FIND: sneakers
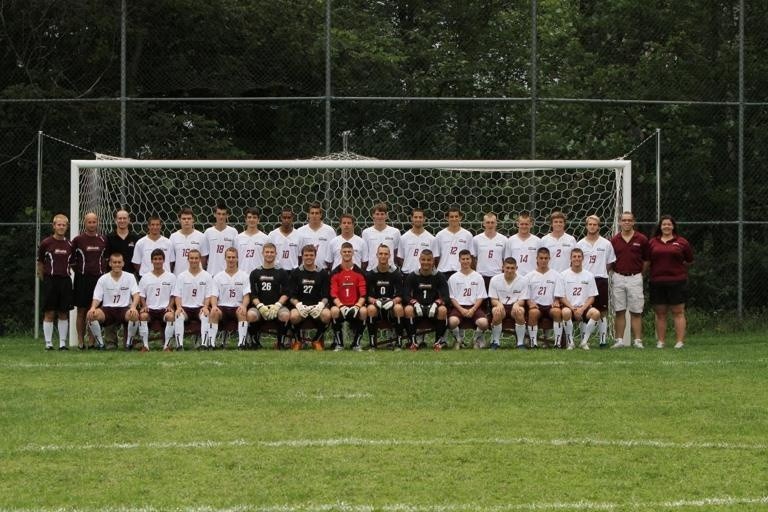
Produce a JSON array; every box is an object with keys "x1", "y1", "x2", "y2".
[
  {"x1": 237, "y1": 343, "x2": 261, "y2": 351},
  {"x1": 196, "y1": 344, "x2": 216, "y2": 352},
  {"x1": 674, "y1": 342, "x2": 685, "y2": 350},
  {"x1": 486, "y1": 343, "x2": 589, "y2": 351},
  {"x1": 633, "y1": 340, "x2": 644, "y2": 350},
  {"x1": 610, "y1": 337, "x2": 624, "y2": 350},
  {"x1": 452, "y1": 340, "x2": 482, "y2": 351},
  {"x1": 58, "y1": 346, "x2": 67, "y2": 352},
  {"x1": 75, "y1": 343, "x2": 119, "y2": 353},
  {"x1": 273, "y1": 337, "x2": 309, "y2": 352},
  {"x1": 312, "y1": 340, "x2": 447, "y2": 354},
  {"x1": 656, "y1": 340, "x2": 664, "y2": 349},
  {"x1": 43, "y1": 345, "x2": 53, "y2": 351},
  {"x1": 123, "y1": 344, "x2": 185, "y2": 354}
]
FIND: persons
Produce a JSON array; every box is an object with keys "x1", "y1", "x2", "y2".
[
  {"x1": 609, "y1": 211, "x2": 651, "y2": 352},
  {"x1": 649, "y1": 215, "x2": 695, "y2": 350},
  {"x1": 70, "y1": 213, "x2": 105, "y2": 351},
  {"x1": 37, "y1": 212, "x2": 74, "y2": 352},
  {"x1": 88, "y1": 202, "x2": 617, "y2": 351}
]
